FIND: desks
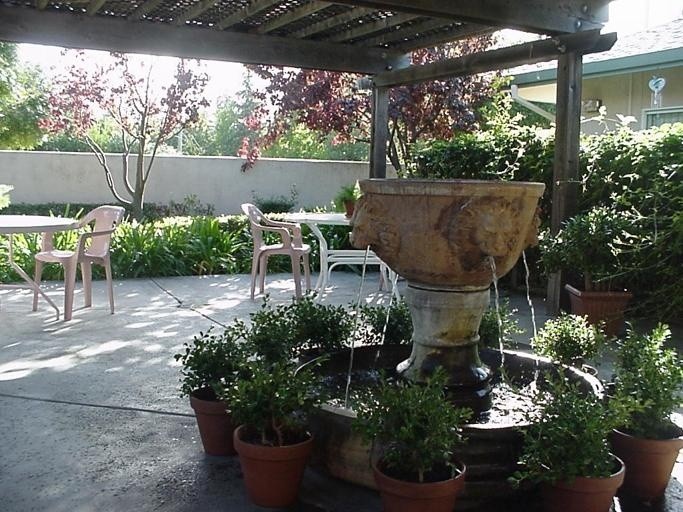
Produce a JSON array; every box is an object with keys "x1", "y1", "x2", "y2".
[
  {"x1": 282, "y1": 210, "x2": 403, "y2": 309},
  {"x1": 0, "y1": 213, "x2": 80, "y2": 323}
]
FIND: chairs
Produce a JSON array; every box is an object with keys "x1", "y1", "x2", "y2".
[
  {"x1": 240, "y1": 201, "x2": 313, "y2": 306},
  {"x1": 30, "y1": 204, "x2": 126, "y2": 321}
]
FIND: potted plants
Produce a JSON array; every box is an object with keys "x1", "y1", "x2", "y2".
[
  {"x1": 341, "y1": 360, "x2": 477, "y2": 512},
  {"x1": 531, "y1": 311, "x2": 602, "y2": 382},
  {"x1": 606, "y1": 321, "x2": 683, "y2": 500},
  {"x1": 211, "y1": 354, "x2": 334, "y2": 508},
  {"x1": 533, "y1": 190, "x2": 648, "y2": 342},
  {"x1": 172, "y1": 323, "x2": 261, "y2": 457},
  {"x1": 497, "y1": 374, "x2": 627, "y2": 512}
]
